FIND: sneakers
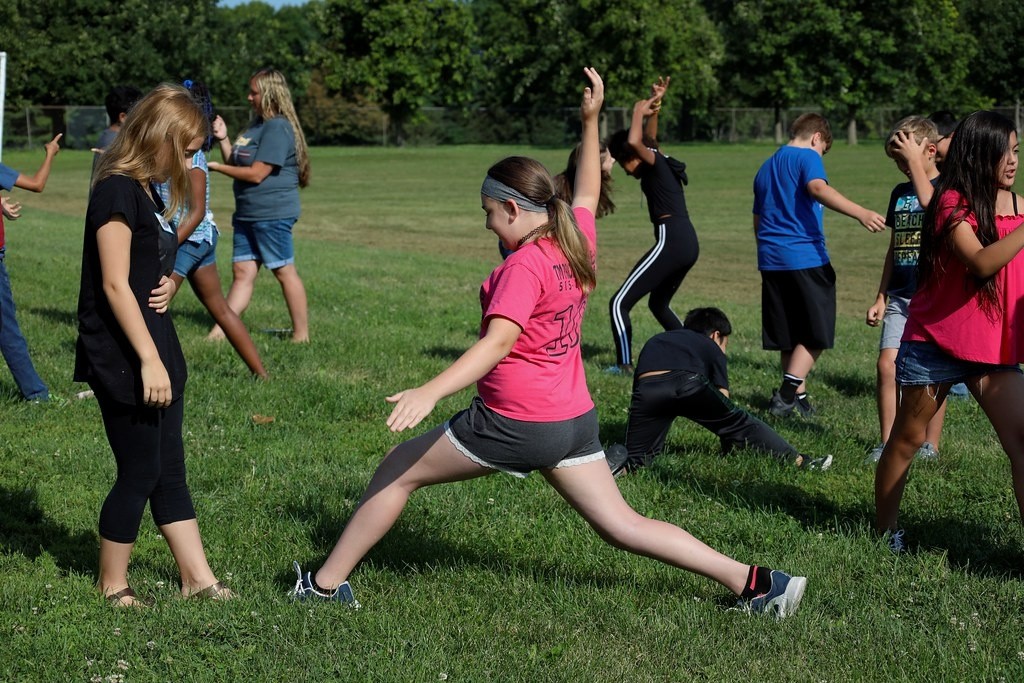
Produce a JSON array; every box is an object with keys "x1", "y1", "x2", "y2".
[
  {"x1": 286, "y1": 560, "x2": 362, "y2": 611},
  {"x1": 604, "y1": 444, "x2": 629, "y2": 480},
  {"x1": 799, "y1": 454, "x2": 833, "y2": 471},
  {"x1": 725, "y1": 570, "x2": 807, "y2": 619},
  {"x1": 768, "y1": 387, "x2": 817, "y2": 421}
]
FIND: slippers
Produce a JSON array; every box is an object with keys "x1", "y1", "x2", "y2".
[
  {"x1": 106, "y1": 587, "x2": 147, "y2": 607},
  {"x1": 188, "y1": 581, "x2": 246, "y2": 601}
]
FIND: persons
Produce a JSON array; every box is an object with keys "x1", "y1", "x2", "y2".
[
  {"x1": 865, "y1": 109, "x2": 1024, "y2": 556},
  {"x1": 603, "y1": 73, "x2": 701, "y2": 373},
  {"x1": 267, "y1": 65, "x2": 808, "y2": 624},
  {"x1": 602, "y1": 306, "x2": 834, "y2": 482},
  {"x1": 752, "y1": 112, "x2": 888, "y2": 420},
  {"x1": 497, "y1": 137, "x2": 615, "y2": 262},
  {"x1": 0, "y1": 132, "x2": 65, "y2": 408},
  {"x1": 69, "y1": 77, "x2": 250, "y2": 620},
  {"x1": 91, "y1": 65, "x2": 309, "y2": 380}
]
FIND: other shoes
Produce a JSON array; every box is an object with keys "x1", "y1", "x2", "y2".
[
  {"x1": 881, "y1": 529, "x2": 906, "y2": 556},
  {"x1": 914, "y1": 442, "x2": 940, "y2": 459},
  {"x1": 604, "y1": 365, "x2": 636, "y2": 373},
  {"x1": 30, "y1": 393, "x2": 67, "y2": 408},
  {"x1": 865, "y1": 443, "x2": 885, "y2": 465}
]
